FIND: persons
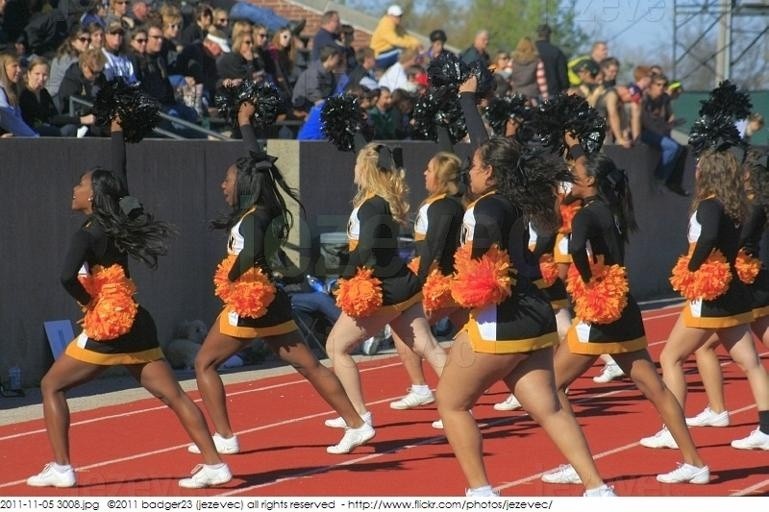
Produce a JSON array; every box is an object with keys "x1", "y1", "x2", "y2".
[
  {"x1": 427, "y1": 49, "x2": 619, "y2": 496},
  {"x1": 27, "y1": 77, "x2": 234, "y2": 488},
  {"x1": 389, "y1": 86, "x2": 475, "y2": 410},
  {"x1": 0, "y1": 0, "x2": 694, "y2": 202},
  {"x1": 483, "y1": 91, "x2": 574, "y2": 411},
  {"x1": 638, "y1": 80, "x2": 769, "y2": 450},
  {"x1": 320, "y1": 94, "x2": 436, "y2": 429},
  {"x1": 188, "y1": 81, "x2": 376, "y2": 455},
  {"x1": 553, "y1": 105, "x2": 626, "y2": 384},
  {"x1": 517, "y1": 93, "x2": 710, "y2": 485}
]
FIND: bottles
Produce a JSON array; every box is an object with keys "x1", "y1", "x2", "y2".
[
  {"x1": 174, "y1": 88, "x2": 182, "y2": 105},
  {"x1": 306, "y1": 274, "x2": 328, "y2": 294}
]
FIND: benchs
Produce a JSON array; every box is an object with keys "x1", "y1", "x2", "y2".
[{"x1": 206, "y1": 117, "x2": 306, "y2": 129}]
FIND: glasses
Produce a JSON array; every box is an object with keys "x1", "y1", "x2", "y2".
[
  {"x1": 500, "y1": 55, "x2": 511, "y2": 60},
  {"x1": 86, "y1": 62, "x2": 105, "y2": 75},
  {"x1": 77, "y1": 1, "x2": 287, "y2": 44}
]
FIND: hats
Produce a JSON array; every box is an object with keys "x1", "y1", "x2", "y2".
[
  {"x1": 105, "y1": 20, "x2": 125, "y2": 34},
  {"x1": 387, "y1": 5, "x2": 404, "y2": 17},
  {"x1": 430, "y1": 29, "x2": 446, "y2": 44},
  {"x1": 206, "y1": 34, "x2": 232, "y2": 54}
]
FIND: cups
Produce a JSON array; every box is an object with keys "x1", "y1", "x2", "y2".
[{"x1": 8, "y1": 368, "x2": 22, "y2": 390}]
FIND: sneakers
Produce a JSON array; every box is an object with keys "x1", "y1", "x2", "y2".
[
  {"x1": 432, "y1": 394, "x2": 618, "y2": 497},
  {"x1": 731, "y1": 425, "x2": 769, "y2": 450},
  {"x1": 684, "y1": 405, "x2": 730, "y2": 428},
  {"x1": 639, "y1": 423, "x2": 679, "y2": 449},
  {"x1": 593, "y1": 359, "x2": 625, "y2": 384},
  {"x1": 666, "y1": 180, "x2": 689, "y2": 196},
  {"x1": 363, "y1": 324, "x2": 392, "y2": 356},
  {"x1": 324, "y1": 410, "x2": 376, "y2": 454},
  {"x1": 188, "y1": 432, "x2": 240, "y2": 455},
  {"x1": 178, "y1": 463, "x2": 232, "y2": 489},
  {"x1": 26, "y1": 462, "x2": 76, "y2": 488},
  {"x1": 656, "y1": 461, "x2": 711, "y2": 484},
  {"x1": 390, "y1": 384, "x2": 435, "y2": 410}
]
